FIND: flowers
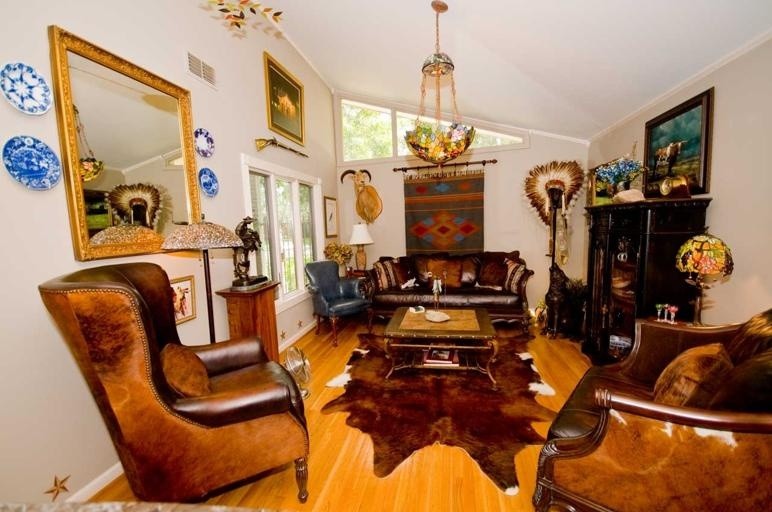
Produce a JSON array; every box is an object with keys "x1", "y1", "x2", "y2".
[
  {"x1": 594, "y1": 156, "x2": 649, "y2": 195},
  {"x1": 324, "y1": 240, "x2": 353, "y2": 264}
]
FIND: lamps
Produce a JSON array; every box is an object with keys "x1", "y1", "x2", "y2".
[
  {"x1": 159, "y1": 212, "x2": 246, "y2": 347},
  {"x1": 70, "y1": 97, "x2": 107, "y2": 184},
  {"x1": 403, "y1": 1, "x2": 476, "y2": 166},
  {"x1": 348, "y1": 222, "x2": 374, "y2": 271},
  {"x1": 676, "y1": 232, "x2": 734, "y2": 328}
]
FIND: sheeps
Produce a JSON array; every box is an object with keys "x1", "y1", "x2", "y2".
[{"x1": 340, "y1": 168, "x2": 383, "y2": 225}]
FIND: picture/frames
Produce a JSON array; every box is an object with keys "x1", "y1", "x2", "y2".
[
  {"x1": 642, "y1": 85, "x2": 716, "y2": 197},
  {"x1": 168, "y1": 273, "x2": 198, "y2": 325},
  {"x1": 324, "y1": 195, "x2": 337, "y2": 237},
  {"x1": 587, "y1": 157, "x2": 626, "y2": 207},
  {"x1": 263, "y1": 50, "x2": 306, "y2": 149}
]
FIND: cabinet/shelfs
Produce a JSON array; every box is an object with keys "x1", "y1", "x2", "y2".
[
  {"x1": 214, "y1": 281, "x2": 281, "y2": 365},
  {"x1": 580, "y1": 196, "x2": 712, "y2": 365}
]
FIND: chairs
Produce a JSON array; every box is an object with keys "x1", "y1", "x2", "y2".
[
  {"x1": 305, "y1": 261, "x2": 371, "y2": 347},
  {"x1": 532, "y1": 308, "x2": 772, "y2": 511},
  {"x1": 38, "y1": 264, "x2": 310, "y2": 506}
]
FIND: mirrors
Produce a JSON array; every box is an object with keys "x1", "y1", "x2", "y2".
[{"x1": 46, "y1": 24, "x2": 206, "y2": 263}]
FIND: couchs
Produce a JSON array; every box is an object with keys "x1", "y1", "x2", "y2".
[{"x1": 366, "y1": 251, "x2": 534, "y2": 337}]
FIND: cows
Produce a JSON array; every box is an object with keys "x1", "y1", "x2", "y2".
[{"x1": 652, "y1": 141, "x2": 688, "y2": 177}]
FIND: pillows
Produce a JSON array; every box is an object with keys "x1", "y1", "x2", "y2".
[
  {"x1": 157, "y1": 342, "x2": 212, "y2": 398},
  {"x1": 652, "y1": 342, "x2": 735, "y2": 411},
  {"x1": 371, "y1": 252, "x2": 526, "y2": 295}
]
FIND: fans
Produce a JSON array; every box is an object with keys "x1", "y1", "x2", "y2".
[{"x1": 281, "y1": 345, "x2": 311, "y2": 401}]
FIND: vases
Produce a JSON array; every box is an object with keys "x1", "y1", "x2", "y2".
[
  {"x1": 338, "y1": 265, "x2": 348, "y2": 278},
  {"x1": 616, "y1": 180, "x2": 630, "y2": 192}
]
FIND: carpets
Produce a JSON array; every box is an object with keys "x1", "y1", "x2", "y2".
[{"x1": 321, "y1": 333, "x2": 558, "y2": 495}]
